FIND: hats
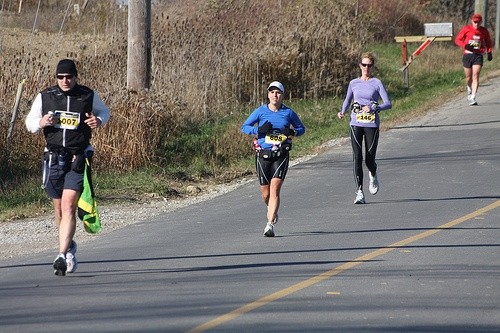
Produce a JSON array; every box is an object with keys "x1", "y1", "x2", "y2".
[
  {"x1": 472, "y1": 14, "x2": 482, "y2": 23},
  {"x1": 266, "y1": 81, "x2": 284, "y2": 94},
  {"x1": 56, "y1": 59, "x2": 78, "y2": 78}
]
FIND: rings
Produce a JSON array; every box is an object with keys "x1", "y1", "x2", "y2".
[{"x1": 46, "y1": 122, "x2": 47, "y2": 124}]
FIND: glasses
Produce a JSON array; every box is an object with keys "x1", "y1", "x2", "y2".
[
  {"x1": 56, "y1": 74, "x2": 74, "y2": 79},
  {"x1": 360, "y1": 62, "x2": 374, "y2": 68}
]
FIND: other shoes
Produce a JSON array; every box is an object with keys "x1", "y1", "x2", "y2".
[
  {"x1": 467, "y1": 85, "x2": 472, "y2": 95},
  {"x1": 470, "y1": 98, "x2": 478, "y2": 105}
]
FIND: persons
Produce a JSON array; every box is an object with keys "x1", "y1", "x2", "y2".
[
  {"x1": 337, "y1": 52, "x2": 392, "y2": 205},
  {"x1": 454, "y1": 14, "x2": 493, "y2": 105},
  {"x1": 25, "y1": 59, "x2": 111, "y2": 277},
  {"x1": 241, "y1": 81, "x2": 305, "y2": 236}
]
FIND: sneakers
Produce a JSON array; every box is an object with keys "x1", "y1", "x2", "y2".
[
  {"x1": 52, "y1": 253, "x2": 67, "y2": 276},
  {"x1": 65, "y1": 240, "x2": 78, "y2": 273},
  {"x1": 354, "y1": 185, "x2": 365, "y2": 204},
  {"x1": 263, "y1": 222, "x2": 275, "y2": 237},
  {"x1": 368, "y1": 171, "x2": 379, "y2": 195},
  {"x1": 266, "y1": 206, "x2": 278, "y2": 224}
]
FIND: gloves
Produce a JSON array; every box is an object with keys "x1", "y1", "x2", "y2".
[
  {"x1": 464, "y1": 44, "x2": 474, "y2": 51},
  {"x1": 258, "y1": 120, "x2": 273, "y2": 135},
  {"x1": 280, "y1": 124, "x2": 295, "y2": 137},
  {"x1": 487, "y1": 52, "x2": 492, "y2": 61}
]
